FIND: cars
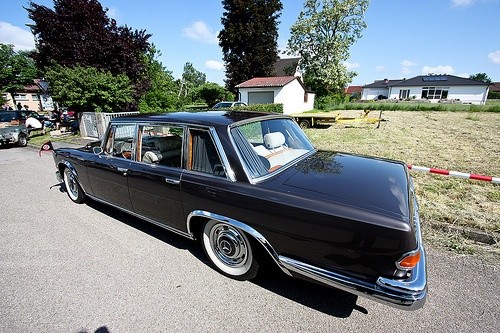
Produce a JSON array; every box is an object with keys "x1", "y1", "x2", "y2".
[
  {"x1": 16, "y1": 110, "x2": 49, "y2": 128},
  {"x1": 53, "y1": 110, "x2": 428, "y2": 311},
  {"x1": 0, "y1": 111, "x2": 27, "y2": 146},
  {"x1": 212, "y1": 101, "x2": 247, "y2": 109}
]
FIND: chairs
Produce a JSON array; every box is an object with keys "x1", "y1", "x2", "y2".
[
  {"x1": 31, "y1": 120, "x2": 45, "y2": 137},
  {"x1": 264, "y1": 132, "x2": 287, "y2": 153}
]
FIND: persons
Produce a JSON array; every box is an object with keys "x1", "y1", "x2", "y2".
[
  {"x1": 44, "y1": 110, "x2": 59, "y2": 127},
  {"x1": 25, "y1": 114, "x2": 42, "y2": 134}
]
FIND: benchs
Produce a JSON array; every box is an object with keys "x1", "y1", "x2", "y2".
[{"x1": 143, "y1": 135, "x2": 182, "y2": 165}]
